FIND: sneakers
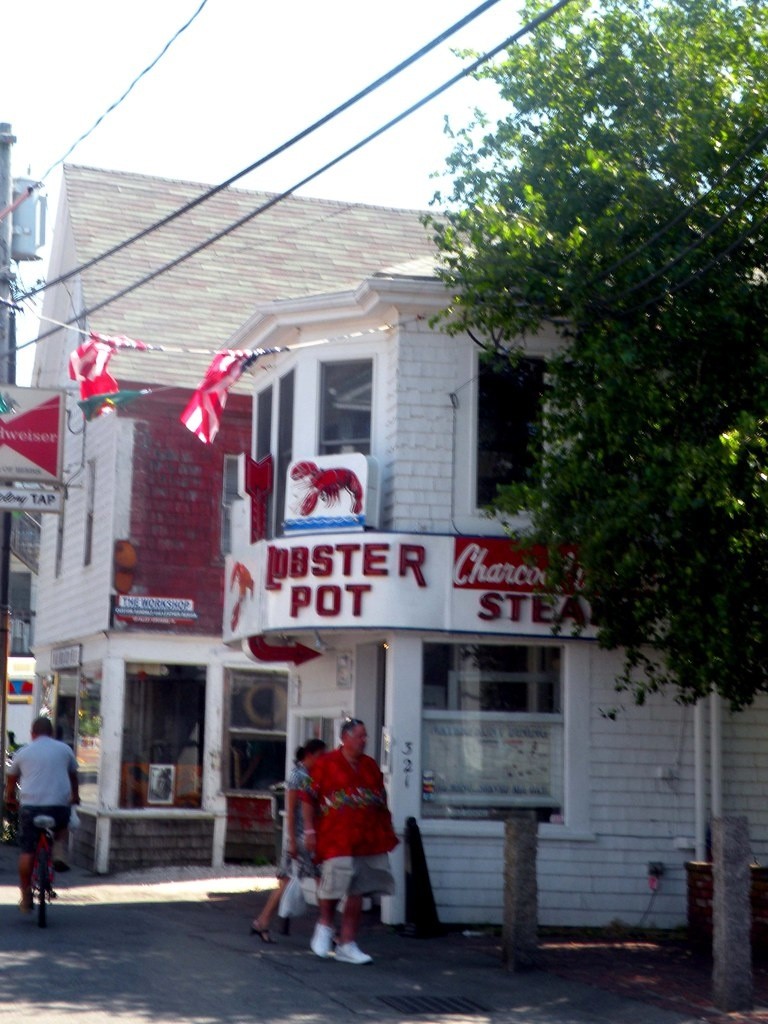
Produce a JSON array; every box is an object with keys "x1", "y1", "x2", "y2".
[
  {"x1": 332, "y1": 940, "x2": 374, "y2": 965},
  {"x1": 307, "y1": 922, "x2": 336, "y2": 959}
]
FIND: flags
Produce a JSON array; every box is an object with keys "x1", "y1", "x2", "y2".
[
  {"x1": 69, "y1": 334, "x2": 162, "y2": 404},
  {"x1": 178, "y1": 346, "x2": 284, "y2": 443},
  {"x1": 80, "y1": 384, "x2": 156, "y2": 422}
]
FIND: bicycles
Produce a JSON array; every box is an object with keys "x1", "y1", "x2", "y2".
[{"x1": 7, "y1": 796, "x2": 82, "y2": 929}]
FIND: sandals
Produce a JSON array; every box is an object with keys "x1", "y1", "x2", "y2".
[{"x1": 252, "y1": 919, "x2": 280, "y2": 945}]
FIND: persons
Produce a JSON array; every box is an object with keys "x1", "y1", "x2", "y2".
[
  {"x1": 251, "y1": 737, "x2": 326, "y2": 942},
  {"x1": 7, "y1": 729, "x2": 20, "y2": 750},
  {"x1": 2, "y1": 716, "x2": 81, "y2": 911},
  {"x1": 300, "y1": 714, "x2": 400, "y2": 965}
]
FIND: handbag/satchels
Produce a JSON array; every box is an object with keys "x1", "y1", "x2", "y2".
[
  {"x1": 67, "y1": 803, "x2": 82, "y2": 835},
  {"x1": 277, "y1": 857, "x2": 309, "y2": 919}
]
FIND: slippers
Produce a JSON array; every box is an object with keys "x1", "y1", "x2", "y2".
[{"x1": 18, "y1": 900, "x2": 32, "y2": 913}]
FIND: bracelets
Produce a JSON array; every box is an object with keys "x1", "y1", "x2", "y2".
[{"x1": 303, "y1": 828, "x2": 316, "y2": 837}]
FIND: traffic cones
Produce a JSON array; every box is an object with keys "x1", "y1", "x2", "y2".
[{"x1": 399, "y1": 817, "x2": 448, "y2": 940}]
